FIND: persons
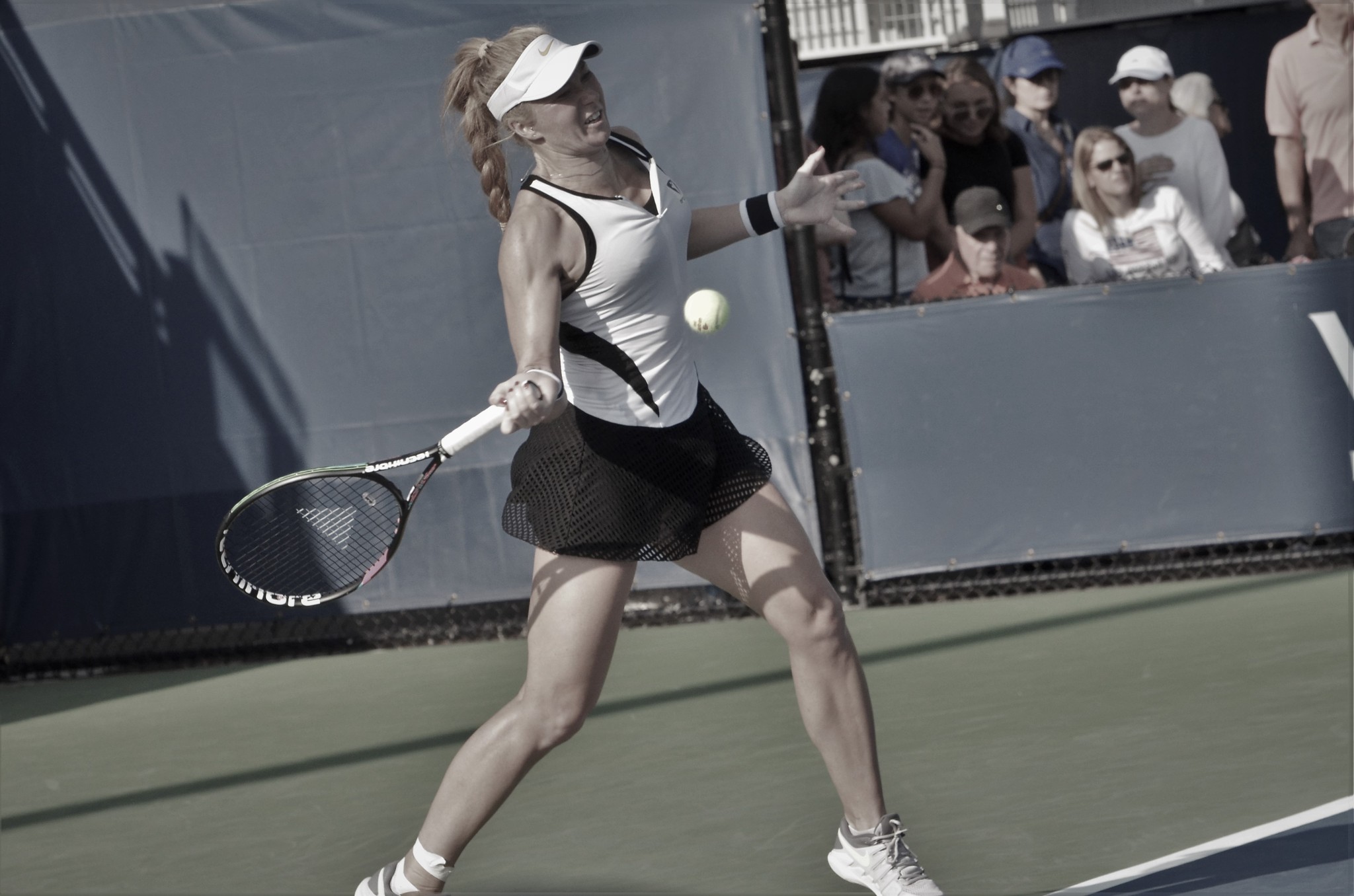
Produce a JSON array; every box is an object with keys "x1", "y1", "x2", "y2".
[
  {"x1": 1265, "y1": 0, "x2": 1353, "y2": 267},
  {"x1": 799, "y1": 35, "x2": 1276, "y2": 312},
  {"x1": 353, "y1": 26, "x2": 941, "y2": 896}
]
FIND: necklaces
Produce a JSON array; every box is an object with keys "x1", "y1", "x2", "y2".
[{"x1": 521, "y1": 149, "x2": 611, "y2": 182}]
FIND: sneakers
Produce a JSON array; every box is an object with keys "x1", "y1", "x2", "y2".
[
  {"x1": 354, "y1": 861, "x2": 399, "y2": 896},
  {"x1": 827, "y1": 813, "x2": 944, "y2": 896}
]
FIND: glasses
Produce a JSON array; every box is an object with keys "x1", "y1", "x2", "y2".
[
  {"x1": 1090, "y1": 152, "x2": 1130, "y2": 170},
  {"x1": 952, "y1": 107, "x2": 992, "y2": 121}
]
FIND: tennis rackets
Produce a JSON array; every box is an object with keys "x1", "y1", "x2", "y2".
[{"x1": 213, "y1": 379, "x2": 545, "y2": 611}]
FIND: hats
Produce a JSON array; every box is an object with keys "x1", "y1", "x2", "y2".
[
  {"x1": 955, "y1": 187, "x2": 1011, "y2": 235},
  {"x1": 881, "y1": 55, "x2": 947, "y2": 83},
  {"x1": 1001, "y1": 37, "x2": 1063, "y2": 78},
  {"x1": 1108, "y1": 45, "x2": 1174, "y2": 82},
  {"x1": 487, "y1": 34, "x2": 603, "y2": 122}
]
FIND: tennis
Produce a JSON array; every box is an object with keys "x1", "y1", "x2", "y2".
[{"x1": 682, "y1": 287, "x2": 731, "y2": 337}]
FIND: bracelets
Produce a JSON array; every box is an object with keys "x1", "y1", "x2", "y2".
[
  {"x1": 738, "y1": 190, "x2": 786, "y2": 237},
  {"x1": 527, "y1": 369, "x2": 562, "y2": 395}
]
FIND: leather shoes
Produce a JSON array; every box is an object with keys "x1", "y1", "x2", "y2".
[{"x1": 903, "y1": 82, "x2": 941, "y2": 99}]
